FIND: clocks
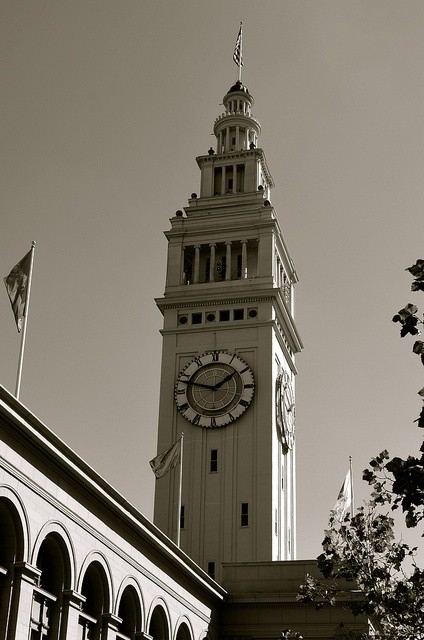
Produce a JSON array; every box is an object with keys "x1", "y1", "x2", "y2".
[
  {"x1": 276, "y1": 371, "x2": 297, "y2": 451},
  {"x1": 174, "y1": 351, "x2": 256, "y2": 430}
]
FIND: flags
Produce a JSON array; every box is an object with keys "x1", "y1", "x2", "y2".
[
  {"x1": 3, "y1": 246, "x2": 34, "y2": 333},
  {"x1": 149, "y1": 437, "x2": 182, "y2": 479},
  {"x1": 328, "y1": 469, "x2": 351, "y2": 523},
  {"x1": 232, "y1": 25, "x2": 242, "y2": 66}
]
